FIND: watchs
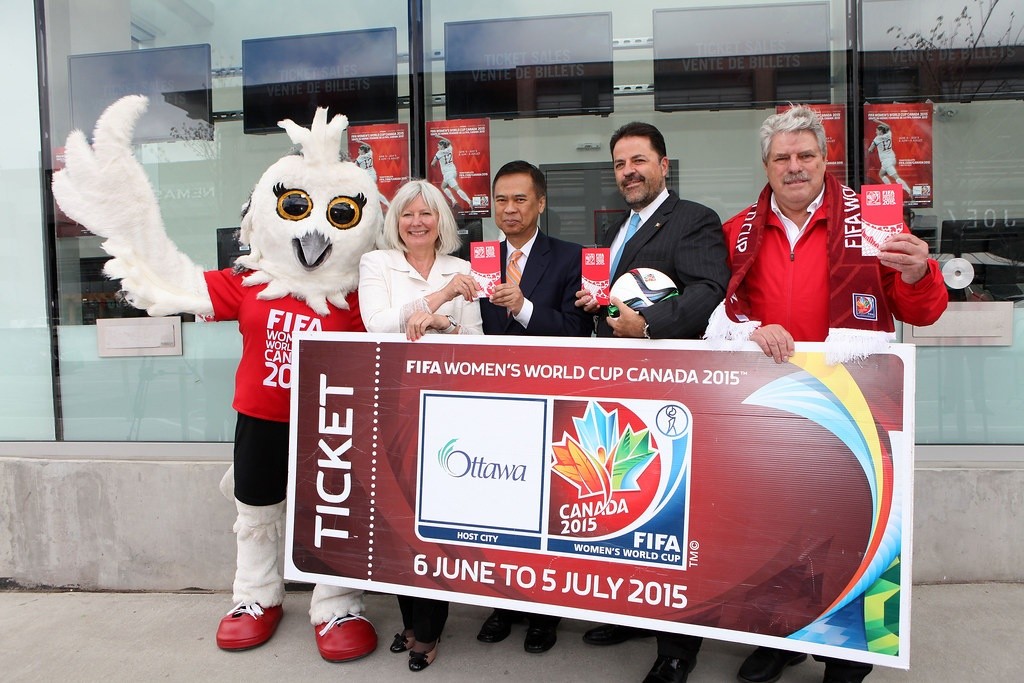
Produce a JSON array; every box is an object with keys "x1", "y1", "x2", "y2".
[{"x1": 437, "y1": 315, "x2": 457, "y2": 334}]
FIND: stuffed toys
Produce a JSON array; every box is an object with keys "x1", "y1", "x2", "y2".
[{"x1": 52, "y1": 96, "x2": 385, "y2": 662}]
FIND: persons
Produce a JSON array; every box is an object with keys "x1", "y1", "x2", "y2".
[
  {"x1": 355, "y1": 139, "x2": 389, "y2": 207},
  {"x1": 576, "y1": 121, "x2": 729, "y2": 683},
  {"x1": 474, "y1": 158, "x2": 587, "y2": 652},
  {"x1": 722, "y1": 109, "x2": 949, "y2": 683},
  {"x1": 869, "y1": 117, "x2": 913, "y2": 198},
  {"x1": 358, "y1": 180, "x2": 480, "y2": 672},
  {"x1": 431, "y1": 134, "x2": 473, "y2": 211}
]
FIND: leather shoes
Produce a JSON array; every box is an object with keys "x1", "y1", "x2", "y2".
[
  {"x1": 524, "y1": 617, "x2": 557, "y2": 654},
  {"x1": 737, "y1": 645, "x2": 807, "y2": 683},
  {"x1": 582, "y1": 624, "x2": 656, "y2": 646},
  {"x1": 641, "y1": 655, "x2": 698, "y2": 683},
  {"x1": 821, "y1": 661, "x2": 874, "y2": 683},
  {"x1": 476, "y1": 608, "x2": 516, "y2": 642}
]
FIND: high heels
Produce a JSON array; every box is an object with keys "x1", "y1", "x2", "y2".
[
  {"x1": 390, "y1": 631, "x2": 416, "y2": 653},
  {"x1": 408, "y1": 635, "x2": 441, "y2": 672}
]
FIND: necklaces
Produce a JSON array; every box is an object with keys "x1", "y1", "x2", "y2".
[{"x1": 418, "y1": 267, "x2": 430, "y2": 276}]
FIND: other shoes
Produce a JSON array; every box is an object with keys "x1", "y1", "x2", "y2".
[
  {"x1": 909, "y1": 190, "x2": 914, "y2": 200},
  {"x1": 452, "y1": 201, "x2": 459, "y2": 209}
]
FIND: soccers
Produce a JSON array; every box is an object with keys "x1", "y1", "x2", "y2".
[{"x1": 606, "y1": 268, "x2": 679, "y2": 319}]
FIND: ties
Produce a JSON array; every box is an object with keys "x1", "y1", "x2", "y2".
[
  {"x1": 505, "y1": 250, "x2": 525, "y2": 317},
  {"x1": 609, "y1": 213, "x2": 640, "y2": 286}
]
